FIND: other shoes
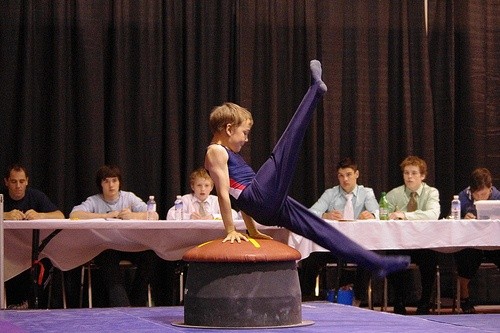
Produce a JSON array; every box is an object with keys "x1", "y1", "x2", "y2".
[
  {"x1": 8, "y1": 301, "x2": 29, "y2": 309},
  {"x1": 359, "y1": 303, "x2": 368, "y2": 307},
  {"x1": 417, "y1": 298, "x2": 430, "y2": 314},
  {"x1": 395, "y1": 302, "x2": 407, "y2": 314},
  {"x1": 461, "y1": 298, "x2": 475, "y2": 313}
]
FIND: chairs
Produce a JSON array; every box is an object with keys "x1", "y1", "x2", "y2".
[
  {"x1": 314, "y1": 261, "x2": 500, "y2": 314},
  {"x1": 79, "y1": 260, "x2": 151, "y2": 307}
]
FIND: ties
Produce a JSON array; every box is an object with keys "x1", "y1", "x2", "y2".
[
  {"x1": 343, "y1": 194, "x2": 355, "y2": 218},
  {"x1": 407, "y1": 192, "x2": 418, "y2": 212},
  {"x1": 199, "y1": 202, "x2": 208, "y2": 217}
]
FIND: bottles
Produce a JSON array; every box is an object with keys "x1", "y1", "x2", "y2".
[
  {"x1": 379, "y1": 191, "x2": 388, "y2": 220},
  {"x1": 175, "y1": 195, "x2": 183, "y2": 221},
  {"x1": 147, "y1": 196, "x2": 157, "y2": 220},
  {"x1": 451, "y1": 195, "x2": 461, "y2": 220}
]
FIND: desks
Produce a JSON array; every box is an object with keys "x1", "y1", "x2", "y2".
[{"x1": 5, "y1": 218, "x2": 500, "y2": 308}]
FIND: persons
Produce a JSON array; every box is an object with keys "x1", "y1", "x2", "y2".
[
  {"x1": 0, "y1": 163, "x2": 65, "y2": 308},
  {"x1": 456, "y1": 167, "x2": 500, "y2": 313},
  {"x1": 203, "y1": 60, "x2": 411, "y2": 282},
  {"x1": 167, "y1": 168, "x2": 227, "y2": 305},
  {"x1": 69, "y1": 165, "x2": 159, "y2": 306},
  {"x1": 299, "y1": 159, "x2": 379, "y2": 306},
  {"x1": 385, "y1": 156, "x2": 440, "y2": 315}
]
